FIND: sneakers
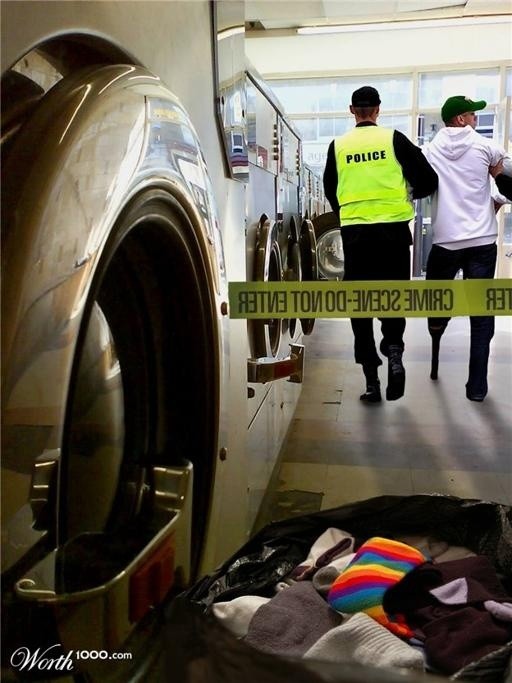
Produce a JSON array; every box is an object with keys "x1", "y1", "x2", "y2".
[{"x1": 465, "y1": 381, "x2": 488, "y2": 401}]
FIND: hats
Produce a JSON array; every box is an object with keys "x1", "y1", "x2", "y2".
[
  {"x1": 441, "y1": 96, "x2": 486, "y2": 121},
  {"x1": 352, "y1": 86, "x2": 381, "y2": 108}
]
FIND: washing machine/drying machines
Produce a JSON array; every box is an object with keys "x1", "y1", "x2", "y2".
[{"x1": 0, "y1": 2, "x2": 345, "y2": 682}]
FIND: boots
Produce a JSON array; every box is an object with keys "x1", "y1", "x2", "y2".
[
  {"x1": 386, "y1": 346, "x2": 405, "y2": 401},
  {"x1": 360, "y1": 362, "x2": 381, "y2": 403}
]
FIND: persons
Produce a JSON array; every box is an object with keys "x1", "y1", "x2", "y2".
[
  {"x1": 322, "y1": 84, "x2": 437, "y2": 402},
  {"x1": 489, "y1": 156, "x2": 511, "y2": 202},
  {"x1": 420, "y1": 95, "x2": 511, "y2": 402}
]
